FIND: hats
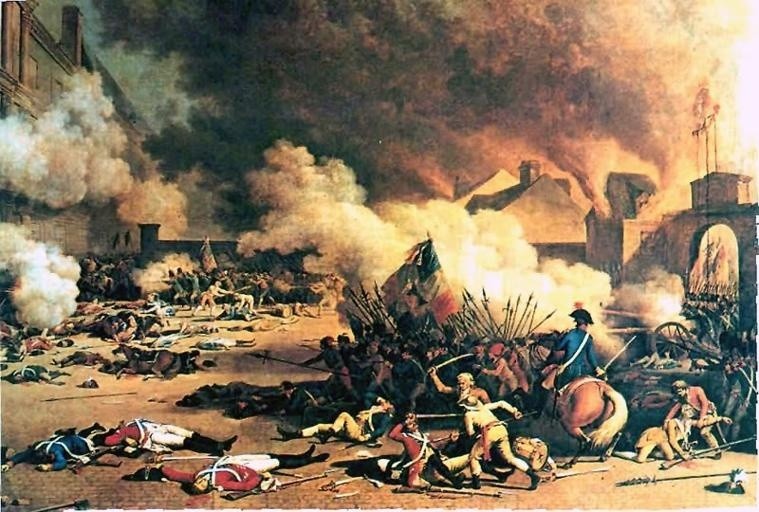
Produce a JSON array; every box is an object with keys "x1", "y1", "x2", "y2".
[
  {"x1": 458, "y1": 397, "x2": 483, "y2": 410},
  {"x1": 568, "y1": 309, "x2": 594, "y2": 324}
]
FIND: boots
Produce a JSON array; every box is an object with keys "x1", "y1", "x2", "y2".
[
  {"x1": 472, "y1": 475, "x2": 481, "y2": 489},
  {"x1": 269, "y1": 445, "x2": 329, "y2": 469},
  {"x1": 430, "y1": 455, "x2": 465, "y2": 489},
  {"x1": 479, "y1": 460, "x2": 515, "y2": 482},
  {"x1": 91, "y1": 428, "x2": 114, "y2": 446},
  {"x1": 313, "y1": 428, "x2": 335, "y2": 444},
  {"x1": 77, "y1": 423, "x2": 105, "y2": 438},
  {"x1": 278, "y1": 427, "x2": 301, "y2": 440},
  {"x1": 526, "y1": 468, "x2": 541, "y2": 490},
  {"x1": 183, "y1": 432, "x2": 237, "y2": 457}
]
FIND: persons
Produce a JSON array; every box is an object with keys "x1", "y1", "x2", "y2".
[{"x1": 3, "y1": 228, "x2": 757, "y2": 511}]
